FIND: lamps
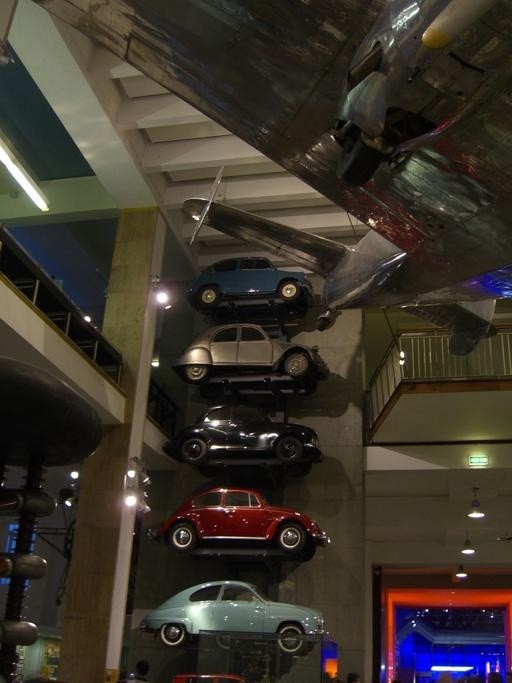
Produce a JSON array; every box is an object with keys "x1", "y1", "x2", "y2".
[
  {"x1": 460, "y1": 527, "x2": 475, "y2": 557},
  {"x1": 138, "y1": 470, "x2": 151, "y2": 484},
  {"x1": 69, "y1": 465, "x2": 79, "y2": 482},
  {"x1": 462, "y1": 495, "x2": 491, "y2": 519},
  {"x1": 126, "y1": 487, "x2": 138, "y2": 507},
  {"x1": 151, "y1": 351, "x2": 160, "y2": 368},
  {"x1": 62, "y1": 491, "x2": 78, "y2": 505},
  {"x1": 0, "y1": 123, "x2": 55, "y2": 214},
  {"x1": 141, "y1": 500, "x2": 152, "y2": 517},
  {"x1": 454, "y1": 559, "x2": 471, "y2": 579},
  {"x1": 125, "y1": 458, "x2": 140, "y2": 480},
  {"x1": 150, "y1": 280, "x2": 180, "y2": 312}
]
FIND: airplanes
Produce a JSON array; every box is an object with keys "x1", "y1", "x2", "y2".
[{"x1": 30, "y1": 0, "x2": 512, "y2": 358}]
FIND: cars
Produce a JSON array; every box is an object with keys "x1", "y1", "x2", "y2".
[
  {"x1": 182, "y1": 256, "x2": 314, "y2": 322},
  {"x1": 172, "y1": 674, "x2": 248, "y2": 683},
  {"x1": 138, "y1": 576, "x2": 329, "y2": 656},
  {"x1": 147, "y1": 483, "x2": 331, "y2": 565},
  {"x1": 169, "y1": 321, "x2": 330, "y2": 398},
  {"x1": 162, "y1": 401, "x2": 325, "y2": 479}
]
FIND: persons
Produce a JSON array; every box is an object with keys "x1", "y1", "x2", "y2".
[
  {"x1": 127, "y1": 658, "x2": 151, "y2": 682},
  {"x1": 346, "y1": 672, "x2": 363, "y2": 683},
  {"x1": 438, "y1": 670, "x2": 511, "y2": 682}
]
